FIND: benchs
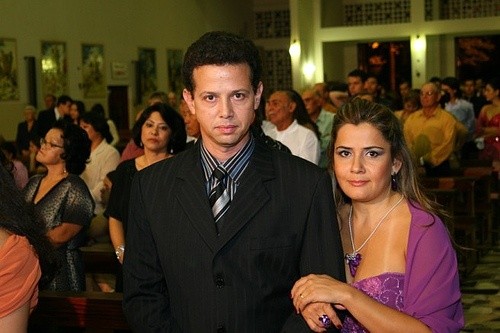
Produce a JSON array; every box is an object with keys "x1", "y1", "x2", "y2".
[
  {"x1": 420, "y1": 158, "x2": 500, "y2": 267},
  {"x1": 29, "y1": 243, "x2": 129, "y2": 333}
]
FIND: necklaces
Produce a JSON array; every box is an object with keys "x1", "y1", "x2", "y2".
[{"x1": 343, "y1": 194, "x2": 404, "y2": 276}]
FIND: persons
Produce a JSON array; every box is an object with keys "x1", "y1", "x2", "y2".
[{"x1": 0, "y1": 31, "x2": 500, "y2": 333}]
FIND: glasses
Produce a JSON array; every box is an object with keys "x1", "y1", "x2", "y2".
[
  {"x1": 39, "y1": 137, "x2": 64, "y2": 150},
  {"x1": 420, "y1": 90, "x2": 437, "y2": 96}
]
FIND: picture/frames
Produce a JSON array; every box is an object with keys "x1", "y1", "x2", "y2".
[
  {"x1": 41, "y1": 39, "x2": 70, "y2": 108},
  {"x1": 81, "y1": 41, "x2": 106, "y2": 98},
  {"x1": 136, "y1": 44, "x2": 156, "y2": 103},
  {"x1": 166, "y1": 49, "x2": 185, "y2": 94},
  {"x1": 0, "y1": 36, "x2": 19, "y2": 101}
]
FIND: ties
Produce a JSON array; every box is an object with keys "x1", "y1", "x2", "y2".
[{"x1": 209, "y1": 166, "x2": 231, "y2": 233}]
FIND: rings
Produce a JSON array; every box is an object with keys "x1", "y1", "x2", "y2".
[{"x1": 319, "y1": 315, "x2": 332, "y2": 327}]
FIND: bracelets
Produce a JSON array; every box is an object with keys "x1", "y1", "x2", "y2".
[{"x1": 115, "y1": 245, "x2": 125, "y2": 259}]
